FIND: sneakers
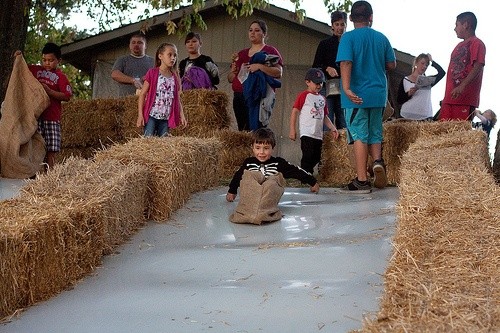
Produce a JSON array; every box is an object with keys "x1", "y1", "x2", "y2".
[
  {"x1": 340, "y1": 176, "x2": 372, "y2": 193},
  {"x1": 372, "y1": 159, "x2": 388, "y2": 188}
]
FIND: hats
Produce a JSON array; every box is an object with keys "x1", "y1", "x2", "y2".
[{"x1": 305, "y1": 68, "x2": 327, "y2": 84}]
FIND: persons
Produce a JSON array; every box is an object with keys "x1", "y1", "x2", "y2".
[
  {"x1": 111, "y1": 33, "x2": 156, "y2": 95},
  {"x1": 13, "y1": 42, "x2": 72, "y2": 173},
  {"x1": 228, "y1": 21, "x2": 284, "y2": 131},
  {"x1": 312, "y1": 11, "x2": 348, "y2": 131},
  {"x1": 397, "y1": 53, "x2": 446, "y2": 121},
  {"x1": 137, "y1": 43, "x2": 187, "y2": 138},
  {"x1": 467, "y1": 109, "x2": 497, "y2": 138},
  {"x1": 335, "y1": 0, "x2": 397, "y2": 192},
  {"x1": 289, "y1": 69, "x2": 338, "y2": 186},
  {"x1": 226, "y1": 129, "x2": 319, "y2": 225},
  {"x1": 438, "y1": 12, "x2": 486, "y2": 121},
  {"x1": 179, "y1": 32, "x2": 220, "y2": 89}
]
